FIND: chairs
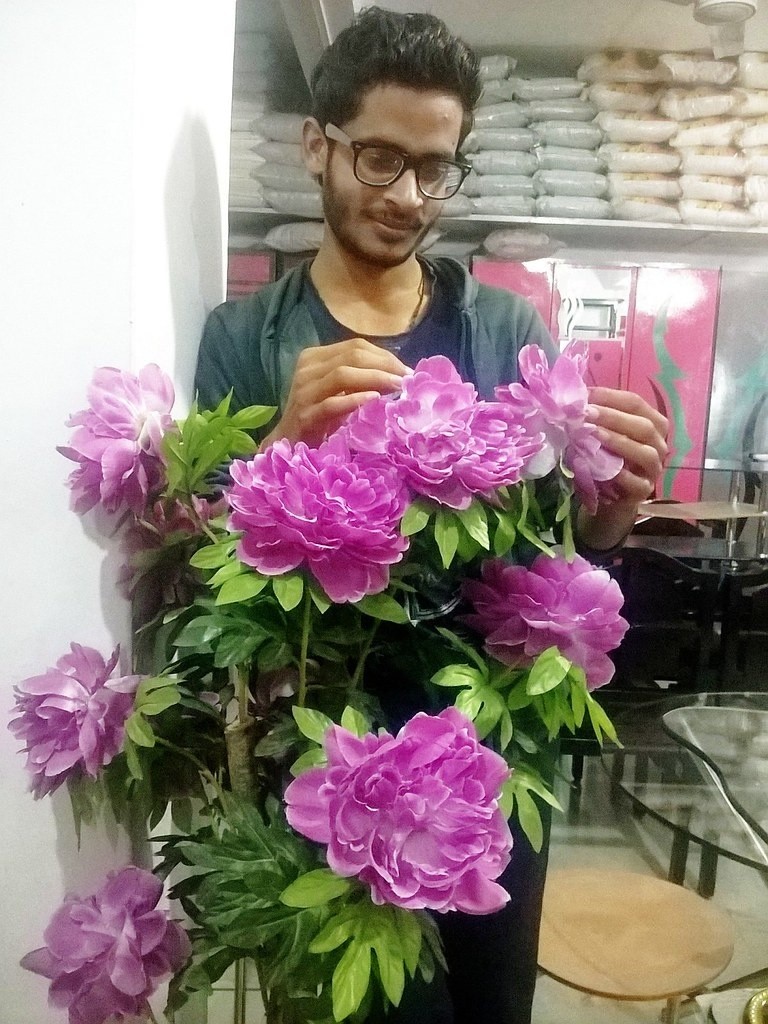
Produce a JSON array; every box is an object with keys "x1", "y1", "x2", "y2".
[
  {"x1": 560, "y1": 547, "x2": 721, "y2": 887},
  {"x1": 657, "y1": 568, "x2": 767, "y2": 899}
]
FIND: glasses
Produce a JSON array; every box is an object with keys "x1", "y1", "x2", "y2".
[{"x1": 325, "y1": 122, "x2": 473, "y2": 200}]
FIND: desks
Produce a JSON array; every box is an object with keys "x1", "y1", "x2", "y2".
[{"x1": 599, "y1": 692, "x2": 768, "y2": 873}]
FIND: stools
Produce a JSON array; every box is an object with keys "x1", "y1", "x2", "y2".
[{"x1": 535, "y1": 866, "x2": 736, "y2": 1024}]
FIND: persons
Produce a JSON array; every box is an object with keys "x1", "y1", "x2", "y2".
[{"x1": 195, "y1": 6, "x2": 668, "y2": 1024}]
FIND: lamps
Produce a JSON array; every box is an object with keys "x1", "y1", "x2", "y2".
[{"x1": 694, "y1": 0, "x2": 758, "y2": 27}]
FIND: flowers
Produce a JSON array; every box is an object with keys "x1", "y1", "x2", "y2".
[{"x1": 9, "y1": 338, "x2": 630, "y2": 1024}]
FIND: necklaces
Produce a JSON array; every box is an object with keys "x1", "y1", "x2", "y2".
[{"x1": 410, "y1": 267, "x2": 425, "y2": 330}]
